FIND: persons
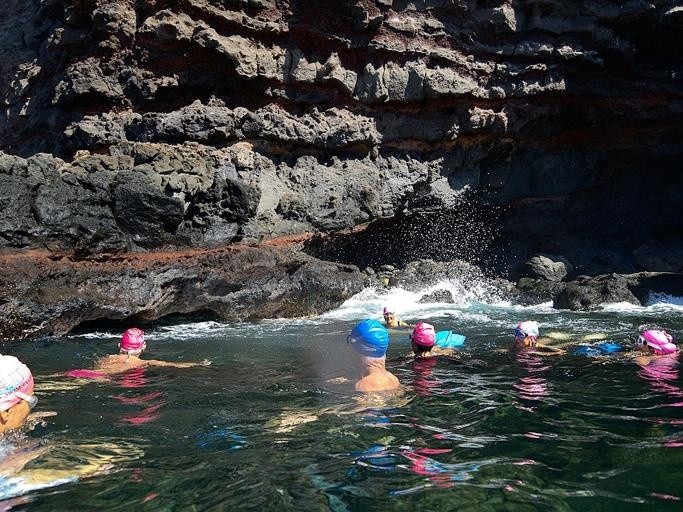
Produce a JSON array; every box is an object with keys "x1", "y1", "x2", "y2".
[
  {"x1": 28, "y1": 326, "x2": 212, "y2": 395},
  {"x1": 590, "y1": 329, "x2": 683, "y2": 365},
  {"x1": 380, "y1": 306, "x2": 409, "y2": 327},
  {"x1": 491, "y1": 321, "x2": 610, "y2": 358},
  {"x1": 261, "y1": 318, "x2": 417, "y2": 432},
  {"x1": 0, "y1": 351, "x2": 143, "y2": 511},
  {"x1": 385, "y1": 321, "x2": 456, "y2": 371}
]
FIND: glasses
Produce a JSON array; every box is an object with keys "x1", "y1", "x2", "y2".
[
  {"x1": 515, "y1": 328, "x2": 528, "y2": 338},
  {"x1": 15, "y1": 392, "x2": 38, "y2": 409},
  {"x1": 638, "y1": 337, "x2": 662, "y2": 351}
]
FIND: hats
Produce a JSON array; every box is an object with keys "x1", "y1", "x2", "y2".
[
  {"x1": 383, "y1": 306, "x2": 394, "y2": 315},
  {"x1": 517, "y1": 320, "x2": 538, "y2": 336},
  {"x1": 120, "y1": 328, "x2": 145, "y2": 350},
  {"x1": 411, "y1": 322, "x2": 434, "y2": 346},
  {"x1": 0, "y1": 353, "x2": 34, "y2": 412},
  {"x1": 350, "y1": 319, "x2": 389, "y2": 358},
  {"x1": 643, "y1": 330, "x2": 676, "y2": 355}
]
